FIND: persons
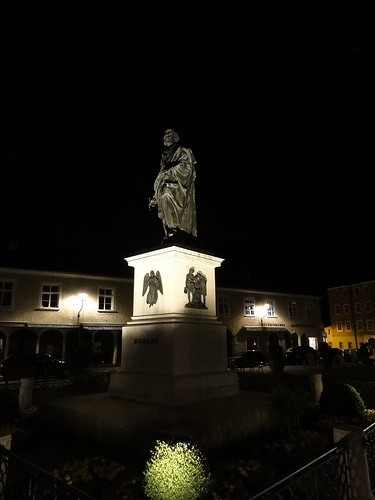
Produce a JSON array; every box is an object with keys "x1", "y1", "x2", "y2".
[
  {"x1": 148, "y1": 129, "x2": 203, "y2": 249},
  {"x1": 196, "y1": 271, "x2": 207, "y2": 307},
  {"x1": 142, "y1": 270, "x2": 163, "y2": 307},
  {"x1": 185, "y1": 267, "x2": 194, "y2": 304}
]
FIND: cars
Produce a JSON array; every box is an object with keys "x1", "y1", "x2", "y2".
[
  {"x1": 226, "y1": 347, "x2": 364, "y2": 369},
  {"x1": 0, "y1": 354, "x2": 75, "y2": 378}
]
